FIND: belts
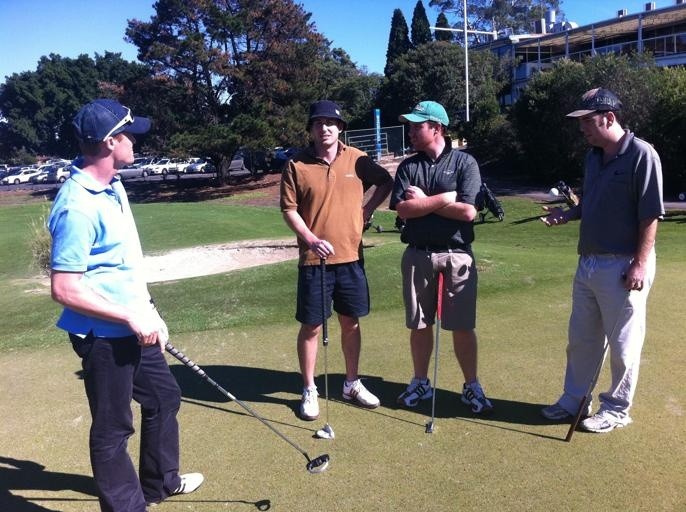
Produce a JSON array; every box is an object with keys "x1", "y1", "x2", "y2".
[
  {"x1": 577, "y1": 245, "x2": 637, "y2": 257},
  {"x1": 407, "y1": 242, "x2": 470, "y2": 255}
]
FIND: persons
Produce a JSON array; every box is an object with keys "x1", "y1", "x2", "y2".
[
  {"x1": 278, "y1": 99, "x2": 393, "y2": 419},
  {"x1": 536, "y1": 87, "x2": 667, "y2": 433},
  {"x1": 385, "y1": 98, "x2": 497, "y2": 414},
  {"x1": 48, "y1": 93, "x2": 204, "y2": 509}
]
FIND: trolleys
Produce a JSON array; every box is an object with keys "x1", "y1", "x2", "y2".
[{"x1": 479, "y1": 182, "x2": 505, "y2": 223}]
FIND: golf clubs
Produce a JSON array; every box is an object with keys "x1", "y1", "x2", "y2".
[
  {"x1": 317, "y1": 257, "x2": 336, "y2": 441},
  {"x1": 426, "y1": 274, "x2": 446, "y2": 433},
  {"x1": 166, "y1": 343, "x2": 332, "y2": 475},
  {"x1": 566, "y1": 272, "x2": 630, "y2": 441}
]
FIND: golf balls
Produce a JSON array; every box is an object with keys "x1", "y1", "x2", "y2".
[{"x1": 549, "y1": 188, "x2": 559, "y2": 198}]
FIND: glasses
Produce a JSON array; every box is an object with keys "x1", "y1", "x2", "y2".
[{"x1": 102, "y1": 104, "x2": 135, "y2": 143}]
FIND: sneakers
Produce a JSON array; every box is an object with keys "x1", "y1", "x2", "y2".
[
  {"x1": 581, "y1": 401, "x2": 633, "y2": 433},
  {"x1": 342, "y1": 379, "x2": 380, "y2": 410},
  {"x1": 541, "y1": 400, "x2": 592, "y2": 422},
  {"x1": 144, "y1": 472, "x2": 204, "y2": 507},
  {"x1": 460, "y1": 381, "x2": 495, "y2": 415},
  {"x1": 396, "y1": 377, "x2": 433, "y2": 408},
  {"x1": 299, "y1": 386, "x2": 320, "y2": 421}
]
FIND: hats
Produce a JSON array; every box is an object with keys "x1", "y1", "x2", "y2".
[
  {"x1": 70, "y1": 97, "x2": 153, "y2": 145},
  {"x1": 564, "y1": 86, "x2": 623, "y2": 118},
  {"x1": 399, "y1": 99, "x2": 450, "y2": 127},
  {"x1": 303, "y1": 99, "x2": 348, "y2": 132}
]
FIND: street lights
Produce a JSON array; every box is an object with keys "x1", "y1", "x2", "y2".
[{"x1": 428, "y1": 26, "x2": 498, "y2": 123}]
